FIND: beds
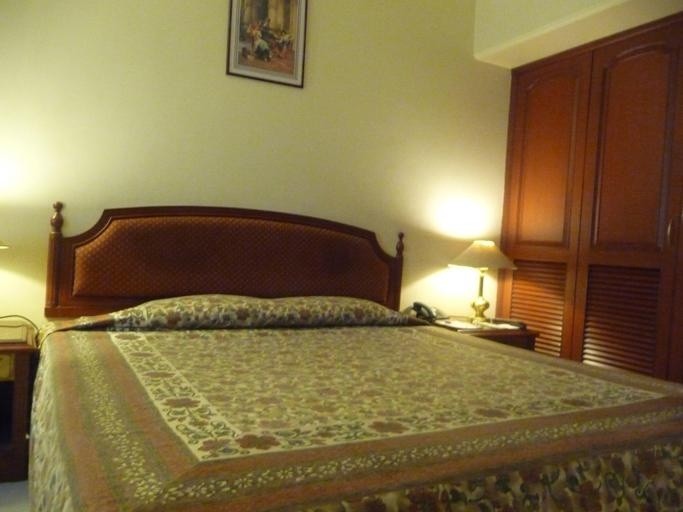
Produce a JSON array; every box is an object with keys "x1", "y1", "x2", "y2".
[{"x1": 27, "y1": 202, "x2": 683, "y2": 512}]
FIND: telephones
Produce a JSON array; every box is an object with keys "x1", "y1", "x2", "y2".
[{"x1": 414, "y1": 302, "x2": 450, "y2": 322}]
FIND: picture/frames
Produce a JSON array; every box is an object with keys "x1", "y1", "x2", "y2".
[{"x1": 225, "y1": 0, "x2": 308, "y2": 88}]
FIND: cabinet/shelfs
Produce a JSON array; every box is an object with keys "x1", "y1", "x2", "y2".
[{"x1": 495, "y1": 9, "x2": 683, "y2": 384}]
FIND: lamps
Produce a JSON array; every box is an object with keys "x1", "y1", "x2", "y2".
[{"x1": 448, "y1": 239, "x2": 518, "y2": 324}]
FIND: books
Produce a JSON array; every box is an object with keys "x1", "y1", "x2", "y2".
[{"x1": 432, "y1": 319, "x2": 483, "y2": 332}]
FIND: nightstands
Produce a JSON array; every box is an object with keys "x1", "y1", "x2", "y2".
[
  {"x1": 434, "y1": 316, "x2": 540, "y2": 352},
  {"x1": 0, "y1": 326, "x2": 39, "y2": 483}
]
